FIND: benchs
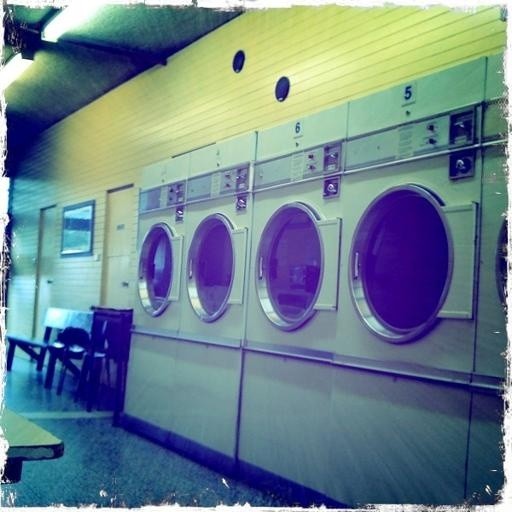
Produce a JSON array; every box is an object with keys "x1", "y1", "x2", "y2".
[{"x1": 7, "y1": 307, "x2": 93, "y2": 390}]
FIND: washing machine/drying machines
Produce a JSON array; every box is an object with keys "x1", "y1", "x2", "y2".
[
  {"x1": 237, "y1": 51, "x2": 505, "y2": 509},
  {"x1": 117, "y1": 129, "x2": 256, "y2": 479}
]
